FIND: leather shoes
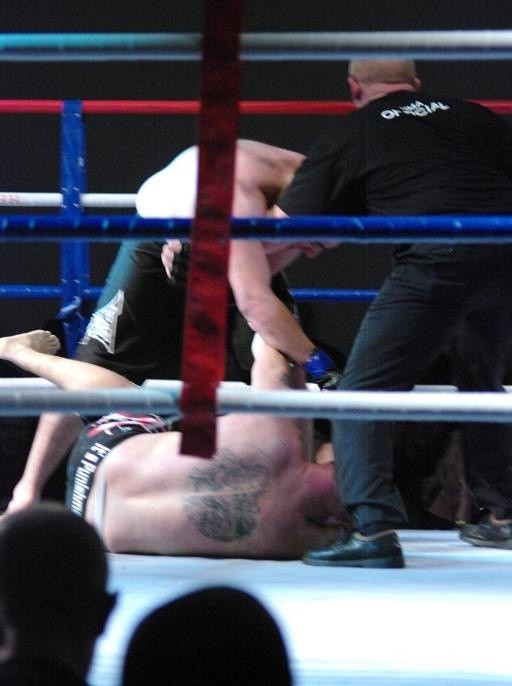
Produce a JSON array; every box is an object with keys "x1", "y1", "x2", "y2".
[
  {"x1": 459, "y1": 513, "x2": 511, "y2": 550},
  {"x1": 301, "y1": 533, "x2": 405, "y2": 568}
]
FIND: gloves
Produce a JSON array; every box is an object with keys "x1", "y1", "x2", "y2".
[{"x1": 302, "y1": 348, "x2": 344, "y2": 389}]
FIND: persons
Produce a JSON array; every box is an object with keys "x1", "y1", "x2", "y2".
[
  {"x1": 161, "y1": 56, "x2": 512, "y2": 568},
  {"x1": 119, "y1": 583, "x2": 294, "y2": 686},
  {"x1": 2, "y1": 499, "x2": 122, "y2": 686},
  {"x1": 3, "y1": 135, "x2": 354, "y2": 536},
  {"x1": 1, "y1": 267, "x2": 354, "y2": 561}
]
